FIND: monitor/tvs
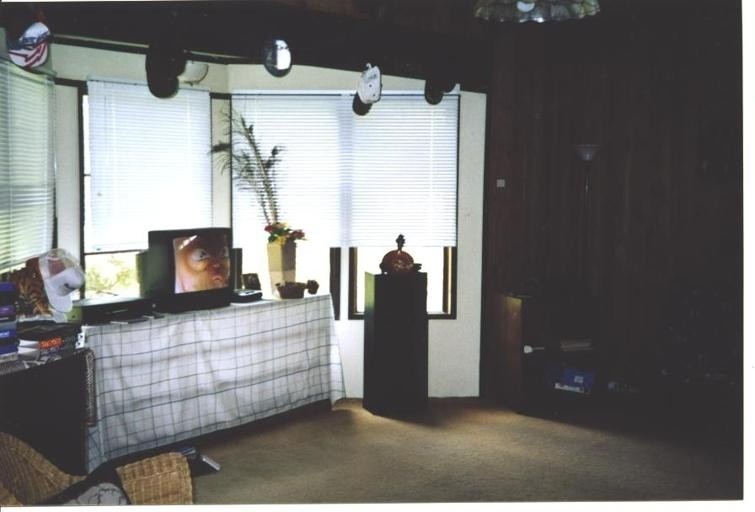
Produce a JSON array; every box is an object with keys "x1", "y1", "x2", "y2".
[{"x1": 137, "y1": 228, "x2": 234, "y2": 314}]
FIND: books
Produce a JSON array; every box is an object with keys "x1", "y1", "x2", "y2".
[
  {"x1": 541, "y1": 329, "x2": 596, "y2": 351},
  {"x1": 0, "y1": 279, "x2": 88, "y2": 370}
]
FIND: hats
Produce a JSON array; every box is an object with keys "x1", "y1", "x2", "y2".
[{"x1": 38, "y1": 246, "x2": 86, "y2": 314}]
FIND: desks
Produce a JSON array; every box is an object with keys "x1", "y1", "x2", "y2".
[{"x1": 76, "y1": 290, "x2": 333, "y2": 481}]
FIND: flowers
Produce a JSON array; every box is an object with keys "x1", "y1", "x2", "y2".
[{"x1": 263, "y1": 220, "x2": 307, "y2": 248}]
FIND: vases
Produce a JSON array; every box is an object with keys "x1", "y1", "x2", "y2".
[{"x1": 266, "y1": 242, "x2": 297, "y2": 297}]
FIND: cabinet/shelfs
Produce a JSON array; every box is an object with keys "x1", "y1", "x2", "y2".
[
  {"x1": 0, "y1": 343, "x2": 90, "y2": 479},
  {"x1": 492, "y1": 294, "x2": 602, "y2": 417}
]
